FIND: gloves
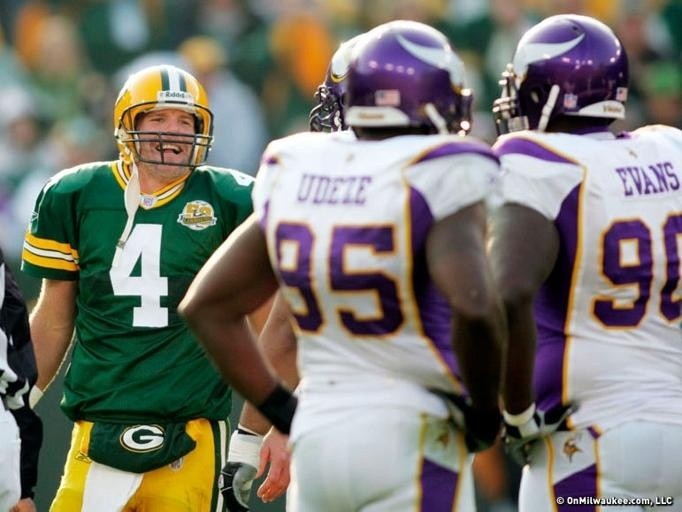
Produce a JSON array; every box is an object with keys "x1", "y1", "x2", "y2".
[
  {"x1": 497, "y1": 395, "x2": 582, "y2": 446},
  {"x1": 214, "y1": 423, "x2": 269, "y2": 512}
]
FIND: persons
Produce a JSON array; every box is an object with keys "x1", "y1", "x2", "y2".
[
  {"x1": 2, "y1": 0, "x2": 680, "y2": 314},
  {"x1": 482, "y1": 11, "x2": 682, "y2": 512},
  {"x1": 216, "y1": 35, "x2": 378, "y2": 512},
  {"x1": 17, "y1": 66, "x2": 302, "y2": 512},
  {"x1": 176, "y1": 15, "x2": 503, "y2": 512},
  {"x1": 0, "y1": 246, "x2": 45, "y2": 510}
]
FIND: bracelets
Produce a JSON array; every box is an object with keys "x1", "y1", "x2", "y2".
[{"x1": 256, "y1": 385, "x2": 298, "y2": 432}]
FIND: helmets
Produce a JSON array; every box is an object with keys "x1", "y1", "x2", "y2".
[
  {"x1": 490, "y1": 12, "x2": 630, "y2": 135},
  {"x1": 310, "y1": 18, "x2": 475, "y2": 141},
  {"x1": 111, "y1": 62, "x2": 216, "y2": 171}
]
